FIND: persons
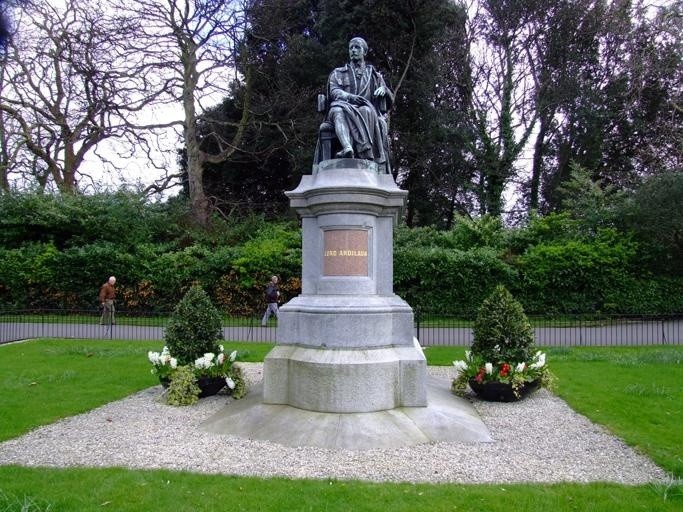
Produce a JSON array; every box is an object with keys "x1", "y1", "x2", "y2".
[
  {"x1": 260, "y1": 275, "x2": 281, "y2": 327},
  {"x1": 98, "y1": 275, "x2": 118, "y2": 325},
  {"x1": 324, "y1": 36, "x2": 397, "y2": 164}
]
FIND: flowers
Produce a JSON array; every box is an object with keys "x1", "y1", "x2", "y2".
[
  {"x1": 146, "y1": 284, "x2": 247, "y2": 406},
  {"x1": 450, "y1": 282, "x2": 559, "y2": 399}
]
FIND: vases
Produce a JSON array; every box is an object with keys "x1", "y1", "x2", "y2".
[
  {"x1": 467, "y1": 378, "x2": 540, "y2": 402},
  {"x1": 158, "y1": 376, "x2": 225, "y2": 398}
]
